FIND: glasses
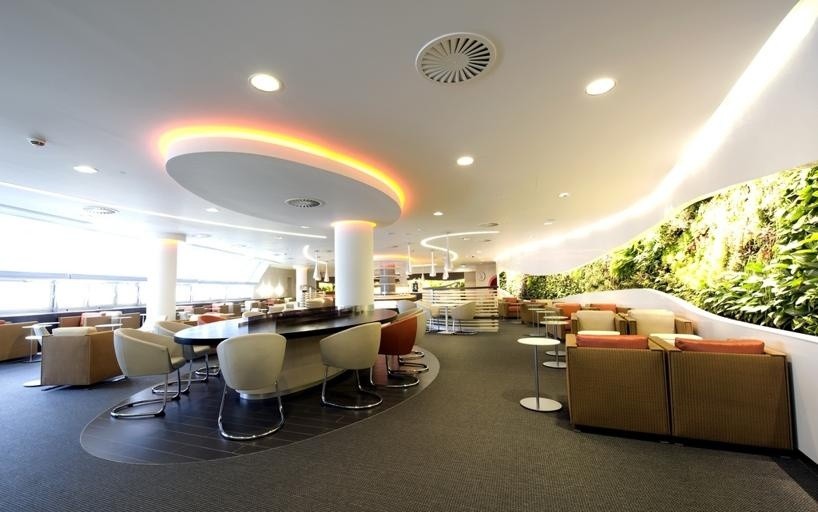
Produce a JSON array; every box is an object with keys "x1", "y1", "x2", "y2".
[{"x1": 247, "y1": 73, "x2": 284, "y2": 95}]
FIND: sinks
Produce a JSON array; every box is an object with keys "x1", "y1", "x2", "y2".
[
  {"x1": 571, "y1": 309, "x2": 628, "y2": 334},
  {"x1": 175, "y1": 297, "x2": 295, "y2": 321},
  {"x1": 648, "y1": 335, "x2": 794, "y2": 450},
  {"x1": 544, "y1": 303, "x2": 581, "y2": 340},
  {"x1": 566, "y1": 333, "x2": 671, "y2": 436},
  {"x1": 112, "y1": 312, "x2": 139, "y2": 329},
  {"x1": 503, "y1": 297, "x2": 524, "y2": 317},
  {"x1": 0, "y1": 321, "x2": 38, "y2": 361},
  {"x1": 40, "y1": 329, "x2": 123, "y2": 390},
  {"x1": 582, "y1": 303, "x2": 632, "y2": 314},
  {"x1": 58, "y1": 312, "x2": 109, "y2": 332},
  {"x1": 618, "y1": 309, "x2": 693, "y2": 334}
]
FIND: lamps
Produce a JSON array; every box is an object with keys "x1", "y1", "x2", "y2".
[
  {"x1": 196, "y1": 315, "x2": 225, "y2": 376},
  {"x1": 157, "y1": 321, "x2": 209, "y2": 393},
  {"x1": 215, "y1": 333, "x2": 287, "y2": 440},
  {"x1": 451, "y1": 302, "x2": 478, "y2": 335},
  {"x1": 370, "y1": 307, "x2": 429, "y2": 388},
  {"x1": 319, "y1": 322, "x2": 383, "y2": 409},
  {"x1": 397, "y1": 300, "x2": 440, "y2": 333},
  {"x1": 110, "y1": 328, "x2": 187, "y2": 418}
]
